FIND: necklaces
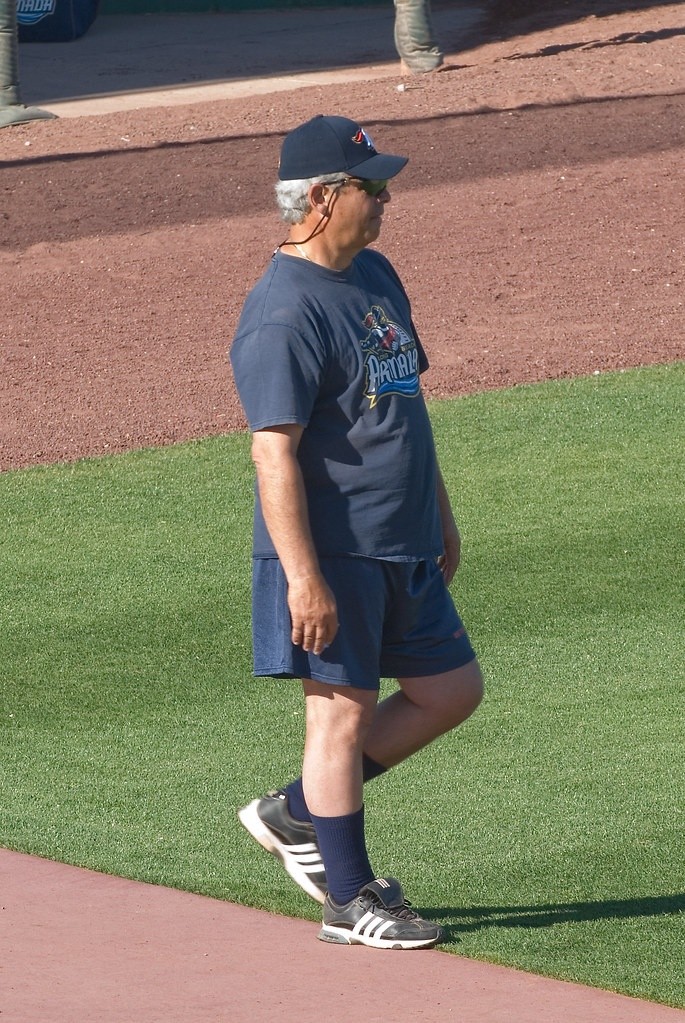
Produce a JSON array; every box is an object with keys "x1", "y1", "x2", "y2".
[{"x1": 294, "y1": 244, "x2": 310, "y2": 261}]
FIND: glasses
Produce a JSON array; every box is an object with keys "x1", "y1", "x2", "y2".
[{"x1": 321, "y1": 176, "x2": 387, "y2": 197}]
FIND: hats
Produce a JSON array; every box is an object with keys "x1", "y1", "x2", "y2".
[{"x1": 279, "y1": 114, "x2": 409, "y2": 181}]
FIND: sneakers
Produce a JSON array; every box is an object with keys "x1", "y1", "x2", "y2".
[
  {"x1": 238, "y1": 787, "x2": 331, "y2": 904},
  {"x1": 319, "y1": 877, "x2": 444, "y2": 950}
]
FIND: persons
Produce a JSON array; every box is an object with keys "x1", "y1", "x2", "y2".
[{"x1": 229, "y1": 113, "x2": 486, "y2": 950}]
